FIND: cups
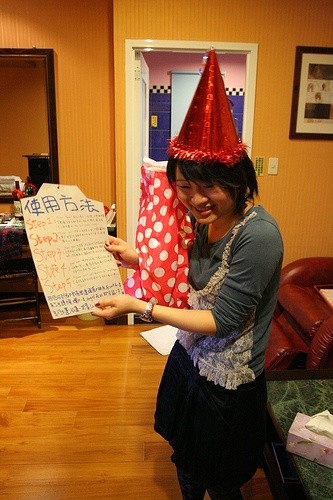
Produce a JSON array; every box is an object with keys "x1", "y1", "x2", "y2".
[
  {"x1": 5, "y1": 212, "x2": 12, "y2": 219},
  {"x1": 0, "y1": 214, "x2": 5, "y2": 220}
]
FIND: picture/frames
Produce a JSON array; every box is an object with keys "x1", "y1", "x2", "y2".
[{"x1": 288, "y1": 46, "x2": 333, "y2": 141}]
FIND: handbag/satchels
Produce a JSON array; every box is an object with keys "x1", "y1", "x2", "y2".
[{"x1": 122, "y1": 157, "x2": 196, "y2": 309}]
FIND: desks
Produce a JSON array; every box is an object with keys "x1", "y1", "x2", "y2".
[{"x1": 259, "y1": 370, "x2": 333, "y2": 500}]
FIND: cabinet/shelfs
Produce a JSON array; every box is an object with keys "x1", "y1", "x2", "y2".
[{"x1": 23, "y1": 154, "x2": 50, "y2": 193}]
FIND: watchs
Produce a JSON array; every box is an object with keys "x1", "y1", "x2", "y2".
[{"x1": 139, "y1": 302, "x2": 153, "y2": 322}]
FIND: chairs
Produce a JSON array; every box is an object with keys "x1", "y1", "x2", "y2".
[{"x1": 0, "y1": 230, "x2": 43, "y2": 331}]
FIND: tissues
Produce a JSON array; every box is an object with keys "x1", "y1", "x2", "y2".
[{"x1": 285, "y1": 409, "x2": 333, "y2": 468}]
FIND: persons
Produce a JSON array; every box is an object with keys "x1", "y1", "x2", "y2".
[{"x1": 91, "y1": 144, "x2": 283, "y2": 500}]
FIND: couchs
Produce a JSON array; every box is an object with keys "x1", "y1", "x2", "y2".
[{"x1": 263, "y1": 256, "x2": 333, "y2": 378}]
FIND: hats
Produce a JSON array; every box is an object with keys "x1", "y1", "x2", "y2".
[{"x1": 166, "y1": 46, "x2": 249, "y2": 168}]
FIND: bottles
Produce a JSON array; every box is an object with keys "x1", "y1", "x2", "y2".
[
  {"x1": 24, "y1": 176, "x2": 34, "y2": 196},
  {"x1": 13, "y1": 181, "x2": 23, "y2": 220}
]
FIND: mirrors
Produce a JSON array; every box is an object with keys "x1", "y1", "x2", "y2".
[{"x1": 0, "y1": 47, "x2": 61, "y2": 203}]
